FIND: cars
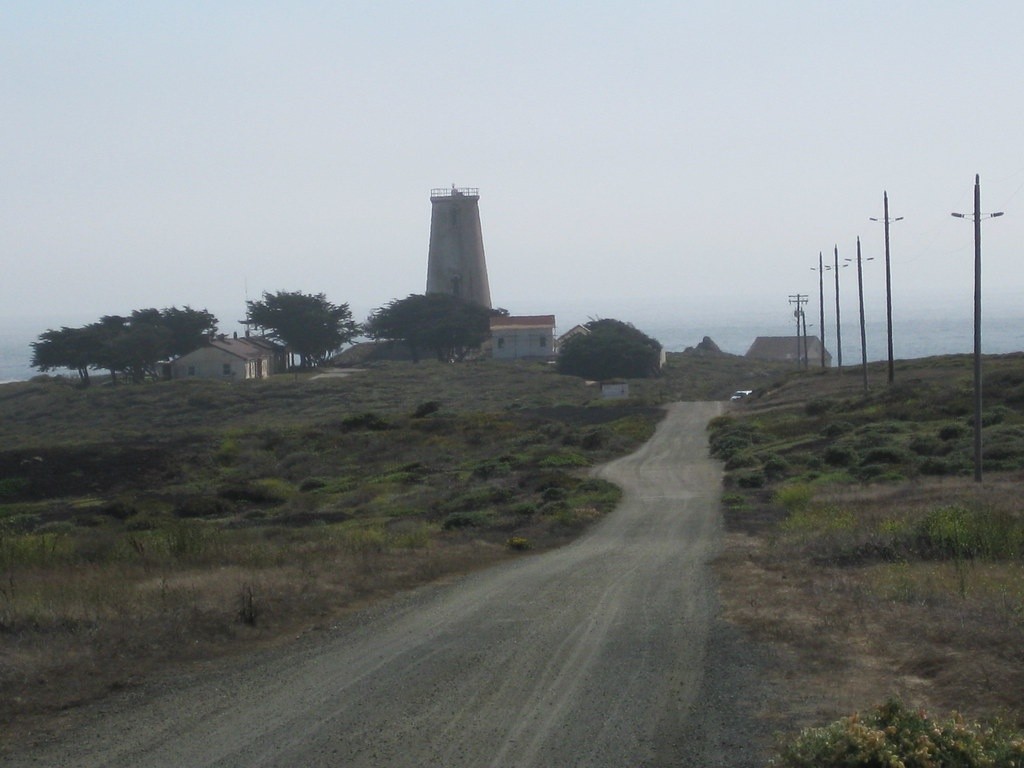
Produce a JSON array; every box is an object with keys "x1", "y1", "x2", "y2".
[{"x1": 730, "y1": 391, "x2": 752, "y2": 401}]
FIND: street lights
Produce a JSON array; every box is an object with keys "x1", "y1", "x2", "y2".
[
  {"x1": 823, "y1": 243, "x2": 849, "y2": 367},
  {"x1": 869, "y1": 190, "x2": 904, "y2": 384},
  {"x1": 845, "y1": 235, "x2": 875, "y2": 396},
  {"x1": 950, "y1": 173, "x2": 1003, "y2": 483},
  {"x1": 810, "y1": 250, "x2": 830, "y2": 369}
]
